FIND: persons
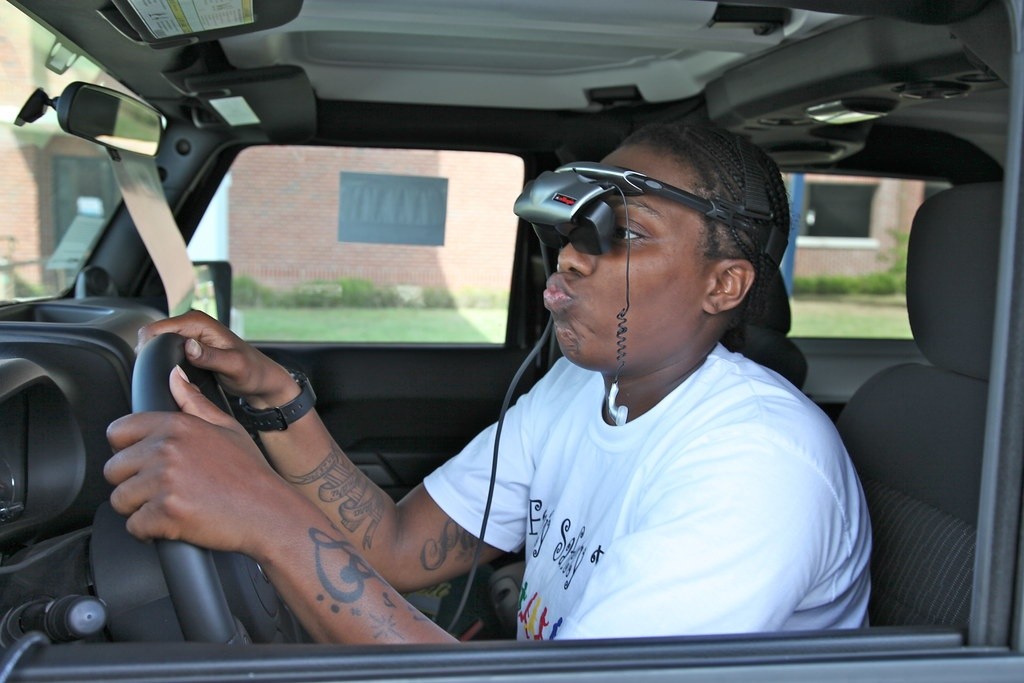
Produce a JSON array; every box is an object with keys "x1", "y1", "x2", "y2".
[{"x1": 104, "y1": 119, "x2": 875, "y2": 644}]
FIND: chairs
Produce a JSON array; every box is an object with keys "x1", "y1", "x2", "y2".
[
  {"x1": 835, "y1": 182, "x2": 1002, "y2": 637},
  {"x1": 721, "y1": 268, "x2": 808, "y2": 391}
]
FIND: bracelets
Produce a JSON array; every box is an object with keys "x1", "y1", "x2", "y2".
[{"x1": 239, "y1": 368, "x2": 317, "y2": 431}]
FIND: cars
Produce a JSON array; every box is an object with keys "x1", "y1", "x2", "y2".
[{"x1": 0, "y1": 0, "x2": 1024, "y2": 680}]
